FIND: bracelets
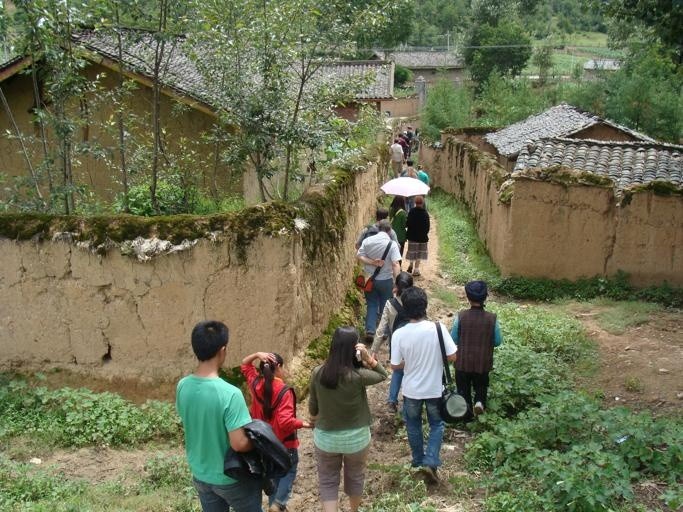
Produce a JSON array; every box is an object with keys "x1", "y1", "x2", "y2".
[{"x1": 368, "y1": 359, "x2": 376, "y2": 367}]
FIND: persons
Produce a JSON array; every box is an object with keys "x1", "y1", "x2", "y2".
[
  {"x1": 355, "y1": 128, "x2": 431, "y2": 339},
  {"x1": 240, "y1": 351, "x2": 307, "y2": 511},
  {"x1": 307, "y1": 326, "x2": 388, "y2": 512},
  {"x1": 388, "y1": 288, "x2": 458, "y2": 484},
  {"x1": 368, "y1": 273, "x2": 414, "y2": 414},
  {"x1": 174, "y1": 321, "x2": 262, "y2": 512},
  {"x1": 451, "y1": 280, "x2": 501, "y2": 418}
]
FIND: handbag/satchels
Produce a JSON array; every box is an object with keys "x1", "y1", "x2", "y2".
[
  {"x1": 440, "y1": 389, "x2": 473, "y2": 423},
  {"x1": 355, "y1": 272, "x2": 372, "y2": 293}
]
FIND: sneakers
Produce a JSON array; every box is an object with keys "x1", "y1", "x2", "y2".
[
  {"x1": 269, "y1": 503, "x2": 288, "y2": 512},
  {"x1": 409, "y1": 464, "x2": 438, "y2": 483},
  {"x1": 407, "y1": 265, "x2": 421, "y2": 275},
  {"x1": 365, "y1": 333, "x2": 374, "y2": 343},
  {"x1": 474, "y1": 401, "x2": 484, "y2": 415}
]
